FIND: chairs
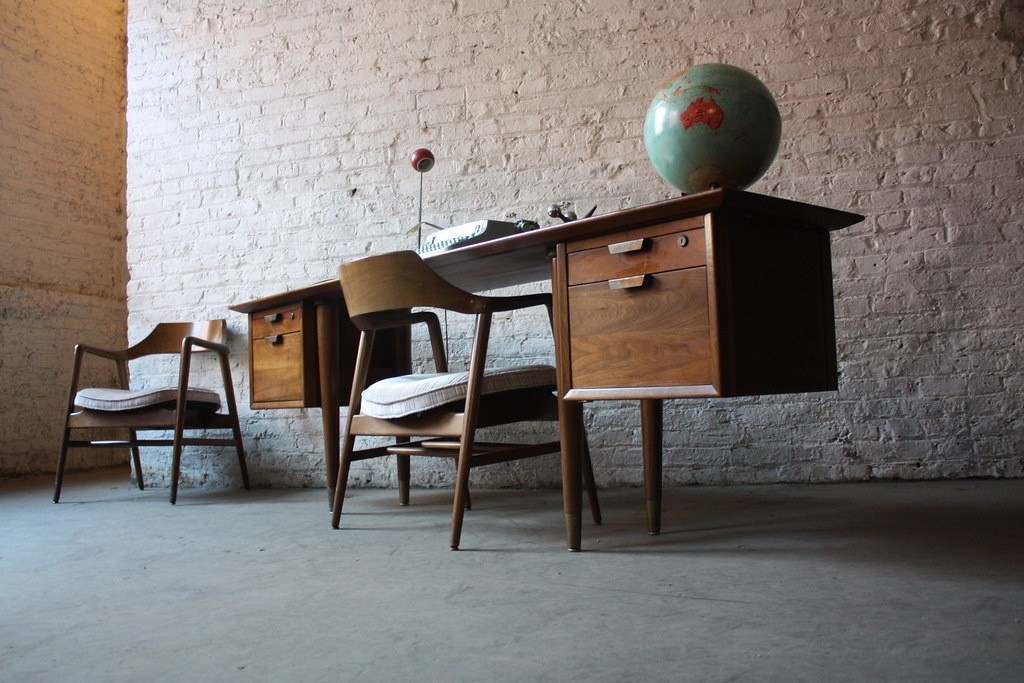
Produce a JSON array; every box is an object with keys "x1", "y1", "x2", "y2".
[
  {"x1": 331, "y1": 249, "x2": 602, "y2": 550},
  {"x1": 53, "y1": 320, "x2": 251, "y2": 505}
]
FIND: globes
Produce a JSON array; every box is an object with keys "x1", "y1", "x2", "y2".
[{"x1": 644, "y1": 63, "x2": 783, "y2": 197}]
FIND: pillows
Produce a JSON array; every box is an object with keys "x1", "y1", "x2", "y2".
[
  {"x1": 72, "y1": 386, "x2": 222, "y2": 412},
  {"x1": 360, "y1": 365, "x2": 556, "y2": 419}
]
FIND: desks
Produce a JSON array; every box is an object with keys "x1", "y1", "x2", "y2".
[{"x1": 226, "y1": 188, "x2": 867, "y2": 537}]
